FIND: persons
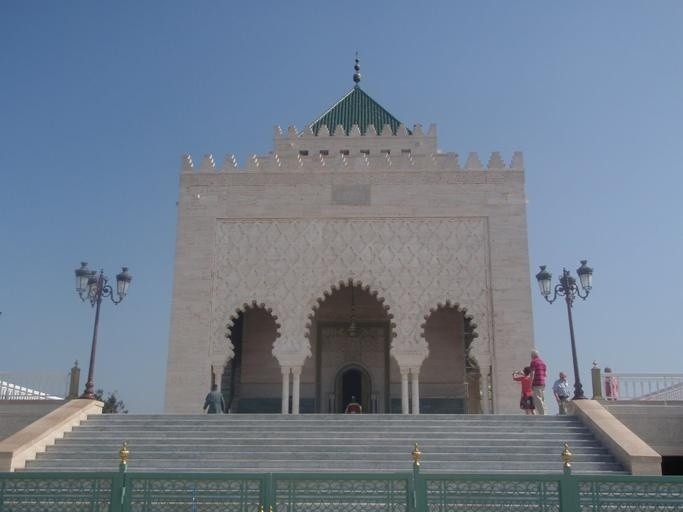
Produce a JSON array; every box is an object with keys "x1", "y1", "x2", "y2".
[
  {"x1": 612, "y1": 376, "x2": 620, "y2": 400},
  {"x1": 512, "y1": 367, "x2": 535, "y2": 414},
  {"x1": 200, "y1": 384, "x2": 228, "y2": 415},
  {"x1": 602, "y1": 368, "x2": 613, "y2": 402},
  {"x1": 552, "y1": 372, "x2": 570, "y2": 414},
  {"x1": 343, "y1": 394, "x2": 362, "y2": 413},
  {"x1": 528, "y1": 350, "x2": 546, "y2": 414}
]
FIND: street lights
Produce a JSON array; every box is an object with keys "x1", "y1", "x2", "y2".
[
  {"x1": 73, "y1": 260, "x2": 134, "y2": 401},
  {"x1": 534, "y1": 258, "x2": 594, "y2": 401}
]
